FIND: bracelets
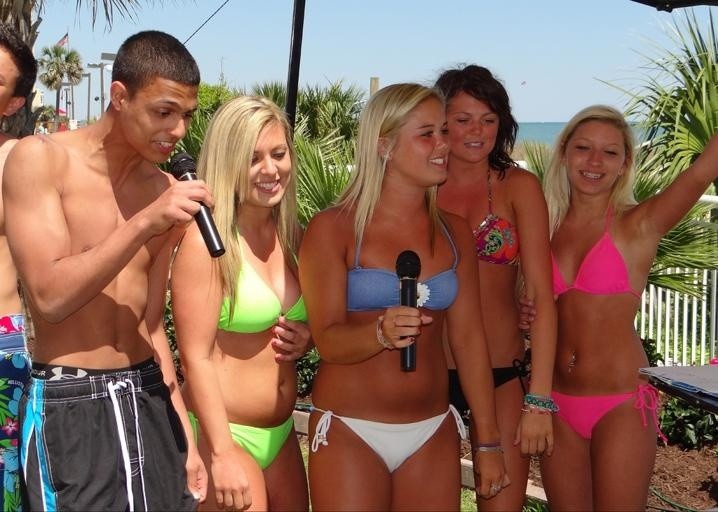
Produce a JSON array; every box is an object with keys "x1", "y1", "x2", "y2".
[
  {"x1": 375, "y1": 315, "x2": 397, "y2": 351},
  {"x1": 472, "y1": 446, "x2": 502, "y2": 453},
  {"x1": 520, "y1": 392, "x2": 560, "y2": 417},
  {"x1": 474, "y1": 441, "x2": 502, "y2": 447}
]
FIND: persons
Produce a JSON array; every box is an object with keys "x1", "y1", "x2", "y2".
[
  {"x1": 430, "y1": 64, "x2": 559, "y2": 511},
  {"x1": 297, "y1": 83, "x2": 510, "y2": 512},
  {"x1": 169, "y1": 96, "x2": 317, "y2": 511},
  {"x1": 517, "y1": 105, "x2": 717, "y2": 512},
  {"x1": 0, "y1": 23, "x2": 39, "y2": 510},
  {"x1": 0, "y1": 30, "x2": 215, "y2": 511}
]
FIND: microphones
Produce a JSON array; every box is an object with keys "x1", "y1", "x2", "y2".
[
  {"x1": 395, "y1": 248, "x2": 421, "y2": 372},
  {"x1": 168, "y1": 153, "x2": 227, "y2": 259}
]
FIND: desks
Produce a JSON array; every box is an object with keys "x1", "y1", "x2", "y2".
[{"x1": 637, "y1": 362, "x2": 718, "y2": 425}]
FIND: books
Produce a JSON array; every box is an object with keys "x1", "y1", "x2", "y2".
[{"x1": 637, "y1": 363, "x2": 718, "y2": 403}]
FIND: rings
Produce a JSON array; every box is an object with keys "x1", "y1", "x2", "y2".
[
  {"x1": 496, "y1": 485, "x2": 501, "y2": 490},
  {"x1": 489, "y1": 482, "x2": 497, "y2": 492}
]
